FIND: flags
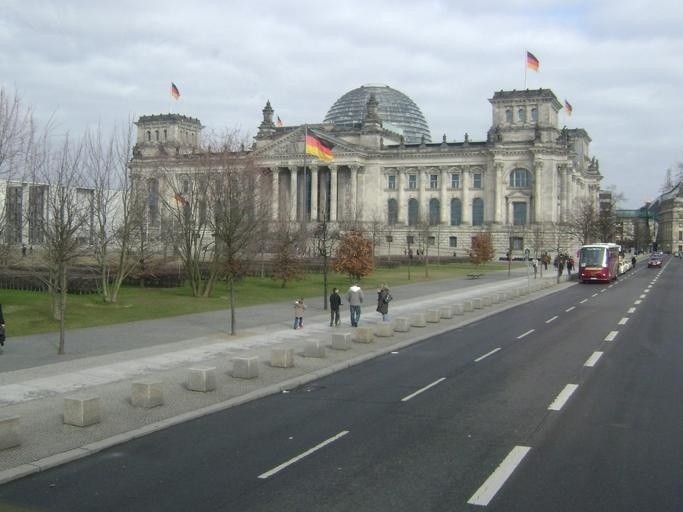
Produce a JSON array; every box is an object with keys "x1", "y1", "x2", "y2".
[
  {"x1": 172, "y1": 83, "x2": 181, "y2": 101},
  {"x1": 306, "y1": 128, "x2": 336, "y2": 161},
  {"x1": 277, "y1": 117, "x2": 284, "y2": 128},
  {"x1": 564, "y1": 100, "x2": 572, "y2": 115},
  {"x1": 173, "y1": 192, "x2": 186, "y2": 202},
  {"x1": 527, "y1": 51, "x2": 538, "y2": 73}
]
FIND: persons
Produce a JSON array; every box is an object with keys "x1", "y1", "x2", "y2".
[
  {"x1": 542, "y1": 252, "x2": 549, "y2": 270},
  {"x1": 532, "y1": 258, "x2": 538, "y2": 273},
  {"x1": 293, "y1": 300, "x2": 307, "y2": 330},
  {"x1": 376, "y1": 285, "x2": 390, "y2": 322},
  {"x1": 329, "y1": 288, "x2": 341, "y2": 327},
  {"x1": 558, "y1": 252, "x2": 575, "y2": 277},
  {"x1": 347, "y1": 280, "x2": 364, "y2": 327},
  {"x1": 631, "y1": 256, "x2": 636, "y2": 268}
]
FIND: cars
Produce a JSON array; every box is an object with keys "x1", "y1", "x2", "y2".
[
  {"x1": 648, "y1": 250, "x2": 663, "y2": 268},
  {"x1": 619, "y1": 260, "x2": 633, "y2": 274}
]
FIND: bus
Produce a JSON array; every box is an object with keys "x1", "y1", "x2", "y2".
[{"x1": 576, "y1": 242, "x2": 622, "y2": 283}]
